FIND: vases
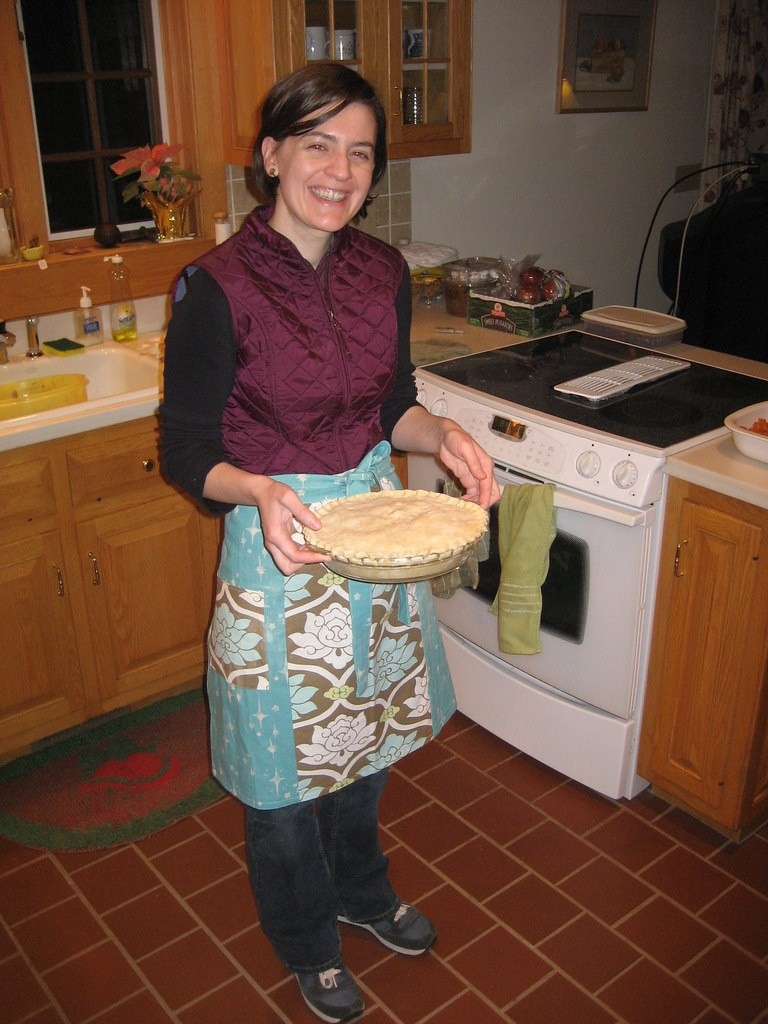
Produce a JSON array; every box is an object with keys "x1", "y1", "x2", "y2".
[{"x1": 140, "y1": 188, "x2": 203, "y2": 238}]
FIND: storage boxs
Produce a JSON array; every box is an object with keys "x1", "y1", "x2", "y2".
[
  {"x1": 465, "y1": 283, "x2": 594, "y2": 339},
  {"x1": 580, "y1": 304, "x2": 687, "y2": 350}
]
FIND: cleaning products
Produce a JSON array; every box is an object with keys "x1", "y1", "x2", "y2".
[
  {"x1": 109, "y1": 253, "x2": 138, "y2": 343},
  {"x1": 73, "y1": 285, "x2": 104, "y2": 347}
]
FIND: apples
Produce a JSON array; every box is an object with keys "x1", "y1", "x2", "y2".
[{"x1": 516, "y1": 267, "x2": 563, "y2": 305}]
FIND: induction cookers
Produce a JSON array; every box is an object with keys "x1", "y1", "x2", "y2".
[{"x1": 413, "y1": 326, "x2": 768, "y2": 507}]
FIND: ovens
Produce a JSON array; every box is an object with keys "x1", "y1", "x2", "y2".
[{"x1": 407, "y1": 452, "x2": 665, "y2": 800}]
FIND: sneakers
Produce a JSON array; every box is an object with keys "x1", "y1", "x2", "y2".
[
  {"x1": 296, "y1": 963, "x2": 365, "y2": 1024},
  {"x1": 338, "y1": 899, "x2": 437, "y2": 955}
]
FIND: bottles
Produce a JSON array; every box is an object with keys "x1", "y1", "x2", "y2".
[{"x1": 104, "y1": 254, "x2": 137, "y2": 342}]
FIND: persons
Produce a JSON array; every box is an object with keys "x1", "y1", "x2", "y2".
[{"x1": 153, "y1": 62, "x2": 502, "y2": 1023}]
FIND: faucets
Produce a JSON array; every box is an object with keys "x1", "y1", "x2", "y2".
[{"x1": 0, "y1": 318, "x2": 16, "y2": 364}]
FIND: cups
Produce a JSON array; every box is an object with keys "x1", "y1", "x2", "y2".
[
  {"x1": 327, "y1": 30, "x2": 357, "y2": 60},
  {"x1": 306, "y1": 27, "x2": 330, "y2": 60},
  {"x1": 403, "y1": 27, "x2": 432, "y2": 61}
]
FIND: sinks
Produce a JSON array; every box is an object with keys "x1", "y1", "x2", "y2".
[{"x1": 0, "y1": 351, "x2": 166, "y2": 428}]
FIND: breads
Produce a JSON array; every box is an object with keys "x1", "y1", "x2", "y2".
[{"x1": 301, "y1": 490, "x2": 490, "y2": 579}]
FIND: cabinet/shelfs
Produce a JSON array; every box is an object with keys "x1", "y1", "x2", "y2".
[
  {"x1": 635, "y1": 476, "x2": 768, "y2": 844},
  {"x1": 223, "y1": 0, "x2": 472, "y2": 170},
  {"x1": 0, "y1": 415, "x2": 226, "y2": 765}
]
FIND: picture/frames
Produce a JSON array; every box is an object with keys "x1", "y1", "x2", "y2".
[{"x1": 555, "y1": 0, "x2": 657, "y2": 115}]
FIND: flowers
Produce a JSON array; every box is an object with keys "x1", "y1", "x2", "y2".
[{"x1": 109, "y1": 142, "x2": 203, "y2": 206}]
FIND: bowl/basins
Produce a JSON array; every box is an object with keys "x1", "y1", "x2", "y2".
[
  {"x1": 303, "y1": 489, "x2": 488, "y2": 584},
  {"x1": 724, "y1": 401, "x2": 768, "y2": 463},
  {"x1": 581, "y1": 305, "x2": 686, "y2": 348},
  {"x1": 442, "y1": 256, "x2": 503, "y2": 318},
  {"x1": 0, "y1": 375, "x2": 90, "y2": 421},
  {"x1": 21, "y1": 245, "x2": 43, "y2": 261}
]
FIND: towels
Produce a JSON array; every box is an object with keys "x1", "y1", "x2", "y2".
[
  {"x1": 430, "y1": 467, "x2": 491, "y2": 600},
  {"x1": 487, "y1": 482, "x2": 558, "y2": 656}
]
